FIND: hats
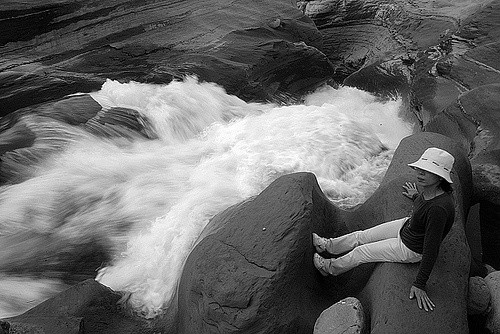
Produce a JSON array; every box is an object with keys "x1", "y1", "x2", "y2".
[{"x1": 407, "y1": 147, "x2": 455, "y2": 183}]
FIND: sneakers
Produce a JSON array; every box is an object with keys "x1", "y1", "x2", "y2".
[
  {"x1": 313, "y1": 252, "x2": 331, "y2": 278},
  {"x1": 312, "y1": 232, "x2": 328, "y2": 254}
]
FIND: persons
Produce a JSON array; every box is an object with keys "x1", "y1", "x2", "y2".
[{"x1": 311, "y1": 147, "x2": 457, "y2": 313}]
